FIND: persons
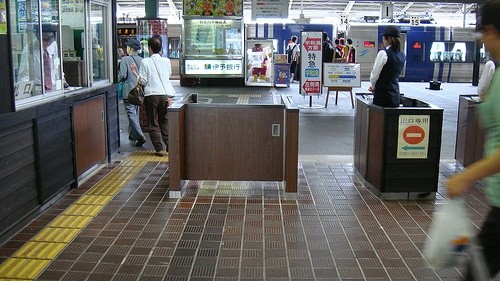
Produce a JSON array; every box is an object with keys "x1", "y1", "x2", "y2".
[
  {"x1": 130, "y1": 35, "x2": 175, "y2": 156},
  {"x1": 476, "y1": 59, "x2": 496, "y2": 100},
  {"x1": 92, "y1": 36, "x2": 102, "y2": 79},
  {"x1": 442, "y1": 0, "x2": 500, "y2": 281},
  {"x1": 18, "y1": 21, "x2": 67, "y2": 92},
  {"x1": 251, "y1": 43, "x2": 267, "y2": 82},
  {"x1": 286, "y1": 35, "x2": 300, "y2": 83},
  {"x1": 116, "y1": 47, "x2": 125, "y2": 59},
  {"x1": 322, "y1": 32, "x2": 356, "y2": 86},
  {"x1": 118, "y1": 39, "x2": 147, "y2": 147},
  {"x1": 368, "y1": 24, "x2": 406, "y2": 108}
]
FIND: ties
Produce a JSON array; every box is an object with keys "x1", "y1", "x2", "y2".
[{"x1": 43, "y1": 48, "x2": 52, "y2": 90}]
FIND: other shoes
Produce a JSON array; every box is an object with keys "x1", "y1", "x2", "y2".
[
  {"x1": 129, "y1": 135, "x2": 134, "y2": 140},
  {"x1": 290, "y1": 79, "x2": 295, "y2": 82},
  {"x1": 135, "y1": 136, "x2": 147, "y2": 146},
  {"x1": 154, "y1": 150, "x2": 166, "y2": 156}
]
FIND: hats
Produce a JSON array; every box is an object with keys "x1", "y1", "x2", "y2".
[
  {"x1": 380, "y1": 25, "x2": 401, "y2": 38},
  {"x1": 125, "y1": 39, "x2": 141, "y2": 50},
  {"x1": 33, "y1": 24, "x2": 57, "y2": 32}
]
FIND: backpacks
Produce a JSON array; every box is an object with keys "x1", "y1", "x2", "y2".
[{"x1": 286, "y1": 44, "x2": 297, "y2": 63}]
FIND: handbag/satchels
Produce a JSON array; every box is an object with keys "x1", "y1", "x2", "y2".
[
  {"x1": 166, "y1": 97, "x2": 172, "y2": 107},
  {"x1": 128, "y1": 84, "x2": 144, "y2": 106},
  {"x1": 422, "y1": 194, "x2": 475, "y2": 267}
]
